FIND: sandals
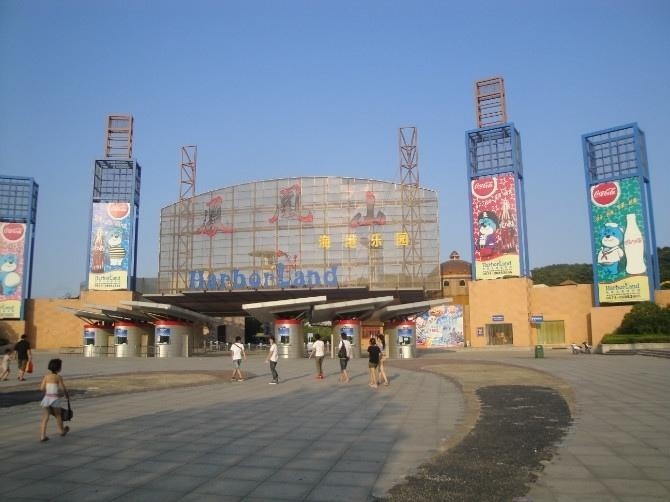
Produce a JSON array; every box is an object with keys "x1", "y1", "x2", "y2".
[{"x1": 369, "y1": 380, "x2": 387, "y2": 387}]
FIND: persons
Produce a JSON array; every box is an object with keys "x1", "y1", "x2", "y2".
[
  {"x1": 308, "y1": 333, "x2": 326, "y2": 379},
  {"x1": 40, "y1": 358, "x2": 70, "y2": 442},
  {"x1": 367, "y1": 337, "x2": 382, "y2": 388},
  {"x1": 266, "y1": 335, "x2": 279, "y2": 384},
  {"x1": 0, "y1": 349, "x2": 13, "y2": 381},
  {"x1": 338, "y1": 332, "x2": 352, "y2": 383},
  {"x1": 375, "y1": 334, "x2": 389, "y2": 386},
  {"x1": 230, "y1": 336, "x2": 246, "y2": 381},
  {"x1": 14, "y1": 334, "x2": 32, "y2": 380}
]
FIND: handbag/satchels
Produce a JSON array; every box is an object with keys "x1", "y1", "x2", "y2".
[
  {"x1": 338, "y1": 346, "x2": 346, "y2": 358},
  {"x1": 50, "y1": 407, "x2": 73, "y2": 421},
  {"x1": 26, "y1": 362, "x2": 33, "y2": 372}
]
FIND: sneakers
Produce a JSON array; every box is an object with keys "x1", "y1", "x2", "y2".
[
  {"x1": 268, "y1": 379, "x2": 279, "y2": 385},
  {"x1": 231, "y1": 376, "x2": 243, "y2": 381}
]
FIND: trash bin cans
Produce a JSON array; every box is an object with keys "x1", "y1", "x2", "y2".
[{"x1": 535, "y1": 345, "x2": 543, "y2": 358}]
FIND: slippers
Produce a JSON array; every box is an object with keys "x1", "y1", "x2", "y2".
[
  {"x1": 41, "y1": 437, "x2": 48, "y2": 440},
  {"x1": 60, "y1": 427, "x2": 69, "y2": 435}
]
